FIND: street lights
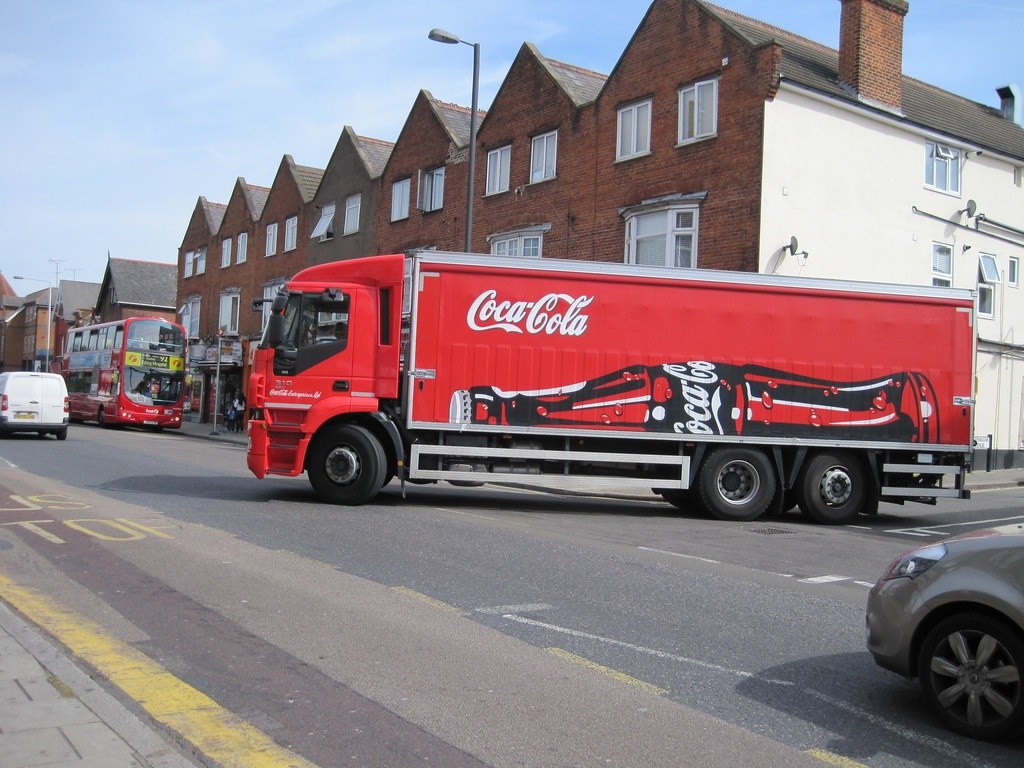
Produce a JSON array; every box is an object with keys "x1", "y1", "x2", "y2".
[
  {"x1": 427, "y1": 27, "x2": 481, "y2": 254},
  {"x1": 13, "y1": 275, "x2": 51, "y2": 374}
]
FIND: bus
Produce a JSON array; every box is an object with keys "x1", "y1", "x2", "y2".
[{"x1": 62, "y1": 316, "x2": 193, "y2": 433}]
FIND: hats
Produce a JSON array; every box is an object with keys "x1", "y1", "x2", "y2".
[{"x1": 236, "y1": 392, "x2": 245, "y2": 400}]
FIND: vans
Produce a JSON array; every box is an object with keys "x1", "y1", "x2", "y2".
[{"x1": 0, "y1": 372, "x2": 70, "y2": 441}]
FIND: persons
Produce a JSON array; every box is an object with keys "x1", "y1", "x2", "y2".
[
  {"x1": 234, "y1": 392, "x2": 246, "y2": 432},
  {"x1": 225, "y1": 403, "x2": 237, "y2": 432},
  {"x1": 334, "y1": 321, "x2": 347, "y2": 341}
]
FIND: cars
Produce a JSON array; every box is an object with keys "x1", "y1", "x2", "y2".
[{"x1": 864, "y1": 524, "x2": 1024, "y2": 745}]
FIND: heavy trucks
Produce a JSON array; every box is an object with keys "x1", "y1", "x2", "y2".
[{"x1": 247, "y1": 247, "x2": 978, "y2": 525}]
